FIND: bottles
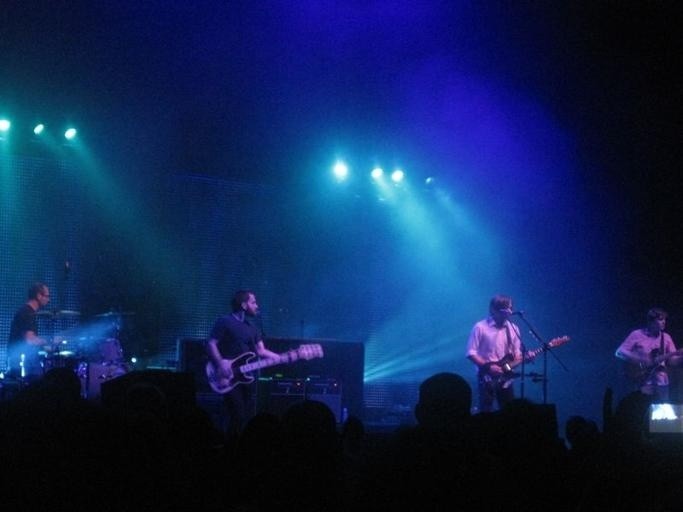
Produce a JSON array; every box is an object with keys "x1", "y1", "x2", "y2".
[{"x1": 342, "y1": 407, "x2": 348, "y2": 423}]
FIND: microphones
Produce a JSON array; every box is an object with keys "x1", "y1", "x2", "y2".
[{"x1": 507, "y1": 310, "x2": 524, "y2": 316}]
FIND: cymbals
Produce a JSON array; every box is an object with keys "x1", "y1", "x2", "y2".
[
  {"x1": 36, "y1": 309, "x2": 80, "y2": 319},
  {"x1": 92, "y1": 311, "x2": 136, "y2": 318}
]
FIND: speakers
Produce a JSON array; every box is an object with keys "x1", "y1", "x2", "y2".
[
  {"x1": 305, "y1": 372, "x2": 342, "y2": 423},
  {"x1": 267, "y1": 378, "x2": 305, "y2": 414}
]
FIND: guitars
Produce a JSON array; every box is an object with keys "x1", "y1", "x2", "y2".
[
  {"x1": 476, "y1": 336, "x2": 569, "y2": 397},
  {"x1": 206, "y1": 344, "x2": 324, "y2": 393},
  {"x1": 624, "y1": 347, "x2": 682, "y2": 385}
]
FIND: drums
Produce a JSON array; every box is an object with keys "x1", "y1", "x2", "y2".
[
  {"x1": 70, "y1": 359, "x2": 128, "y2": 400},
  {"x1": 45, "y1": 351, "x2": 77, "y2": 374},
  {"x1": 80, "y1": 337, "x2": 122, "y2": 358}
]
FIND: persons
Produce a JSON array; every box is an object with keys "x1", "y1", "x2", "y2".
[
  {"x1": 613, "y1": 305, "x2": 683, "y2": 405},
  {"x1": 207, "y1": 289, "x2": 295, "y2": 449},
  {"x1": 7, "y1": 282, "x2": 50, "y2": 379},
  {"x1": 0, "y1": 367, "x2": 683, "y2": 512},
  {"x1": 466, "y1": 294, "x2": 536, "y2": 412}
]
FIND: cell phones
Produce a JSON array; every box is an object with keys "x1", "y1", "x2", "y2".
[{"x1": 647, "y1": 402, "x2": 683, "y2": 435}]
